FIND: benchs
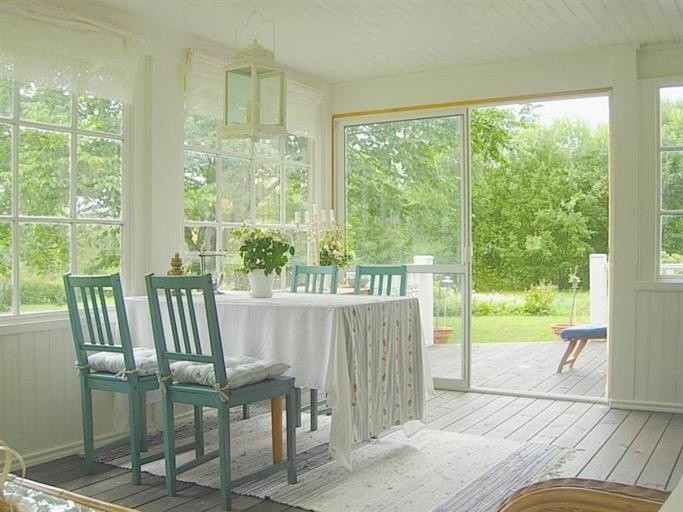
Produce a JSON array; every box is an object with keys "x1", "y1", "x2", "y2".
[{"x1": 557, "y1": 327, "x2": 606, "y2": 373}]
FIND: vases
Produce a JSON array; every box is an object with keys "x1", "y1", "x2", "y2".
[{"x1": 326, "y1": 265, "x2": 344, "y2": 289}]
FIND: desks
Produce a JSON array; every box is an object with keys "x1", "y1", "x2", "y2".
[{"x1": 116, "y1": 292, "x2": 435, "y2": 474}]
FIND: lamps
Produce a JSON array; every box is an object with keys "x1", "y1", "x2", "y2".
[{"x1": 220, "y1": 5, "x2": 287, "y2": 144}]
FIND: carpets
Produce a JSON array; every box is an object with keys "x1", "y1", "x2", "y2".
[
  {"x1": 496, "y1": 477, "x2": 669, "y2": 511},
  {"x1": 76, "y1": 405, "x2": 575, "y2": 512}
]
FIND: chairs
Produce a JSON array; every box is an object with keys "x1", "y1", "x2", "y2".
[
  {"x1": 310, "y1": 265, "x2": 407, "y2": 430},
  {"x1": 63, "y1": 273, "x2": 204, "y2": 485},
  {"x1": 242, "y1": 265, "x2": 338, "y2": 427},
  {"x1": 144, "y1": 273, "x2": 297, "y2": 511}
]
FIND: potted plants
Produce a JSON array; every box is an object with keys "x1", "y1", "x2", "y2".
[
  {"x1": 434, "y1": 280, "x2": 454, "y2": 344},
  {"x1": 551, "y1": 264, "x2": 582, "y2": 335},
  {"x1": 230, "y1": 222, "x2": 295, "y2": 297},
  {"x1": 319, "y1": 230, "x2": 355, "y2": 289}
]
